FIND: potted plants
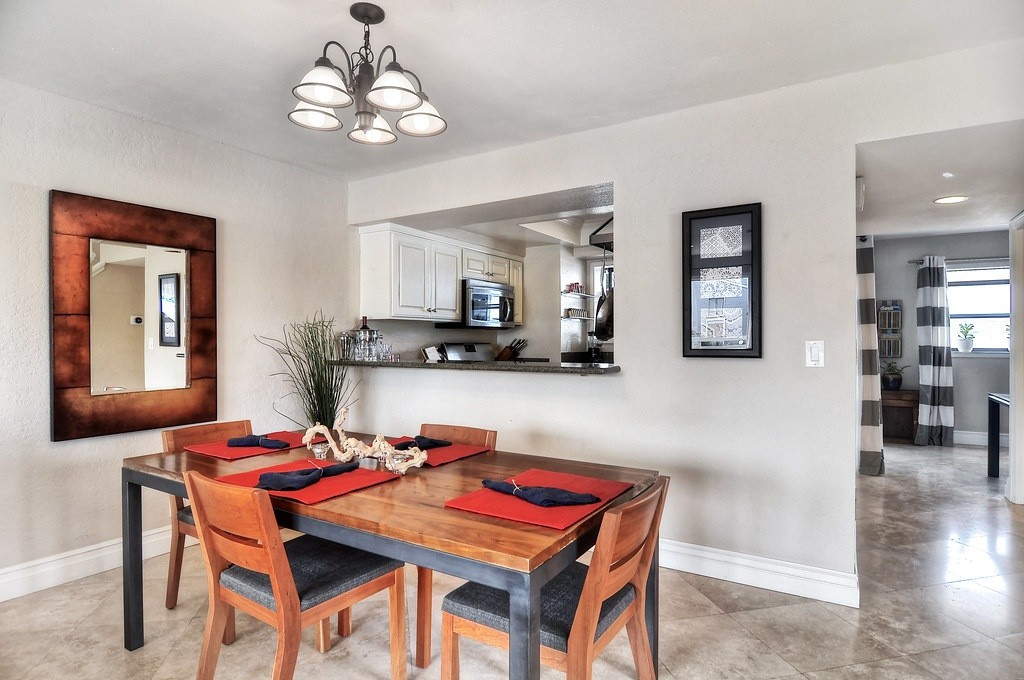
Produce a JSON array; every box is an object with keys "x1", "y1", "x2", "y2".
[
  {"x1": 879, "y1": 360, "x2": 911, "y2": 390},
  {"x1": 957, "y1": 322, "x2": 975, "y2": 353}
]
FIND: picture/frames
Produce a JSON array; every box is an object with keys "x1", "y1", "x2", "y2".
[
  {"x1": 158, "y1": 273, "x2": 179, "y2": 346},
  {"x1": 678, "y1": 202, "x2": 761, "y2": 358},
  {"x1": 48, "y1": 187, "x2": 218, "y2": 444}
]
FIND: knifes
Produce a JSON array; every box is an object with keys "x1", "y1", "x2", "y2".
[{"x1": 508, "y1": 338, "x2": 526, "y2": 353}]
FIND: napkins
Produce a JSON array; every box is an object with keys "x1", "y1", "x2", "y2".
[
  {"x1": 226, "y1": 434, "x2": 291, "y2": 449},
  {"x1": 250, "y1": 462, "x2": 360, "y2": 490},
  {"x1": 482, "y1": 478, "x2": 602, "y2": 506},
  {"x1": 392, "y1": 437, "x2": 453, "y2": 450}
]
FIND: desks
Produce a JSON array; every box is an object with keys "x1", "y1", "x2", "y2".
[
  {"x1": 122, "y1": 428, "x2": 663, "y2": 680},
  {"x1": 987, "y1": 392, "x2": 1010, "y2": 477},
  {"x1": 880, "y1": 388, "x2": 919, "y2": 445}
]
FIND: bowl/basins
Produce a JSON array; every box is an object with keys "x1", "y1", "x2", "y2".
[{"x1": 311, "y1": 443, "x2": 330, "y2": 458}]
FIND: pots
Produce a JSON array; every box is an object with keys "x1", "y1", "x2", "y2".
[{"x1": 595, "y1": 287, "x2": 613, "y2": 341}]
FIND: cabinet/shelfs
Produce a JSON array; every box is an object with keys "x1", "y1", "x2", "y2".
[{"x1": 358, "y1": 223, "x2": 525, "y2": 326}]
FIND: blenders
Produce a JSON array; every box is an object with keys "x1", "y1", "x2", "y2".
[{"x1": 587, "y1": 331, "x2": 602, "y2": 362}]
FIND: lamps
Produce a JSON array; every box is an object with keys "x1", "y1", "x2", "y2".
[{"x1": 288, "y1": 1, "x2": 446, "y2": 144}]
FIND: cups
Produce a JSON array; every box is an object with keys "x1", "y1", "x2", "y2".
[{"x1": 353, "y1": 343, "x2": 392, "y2": 360}]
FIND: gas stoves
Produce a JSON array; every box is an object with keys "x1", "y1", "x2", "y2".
[{"x1": 437, "y1": 341, "x2": 495, "y2": 363}]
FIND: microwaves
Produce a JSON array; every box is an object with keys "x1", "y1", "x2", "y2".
[{"x1": 435, "y1": 278, "x2": 515, "y2": 330}]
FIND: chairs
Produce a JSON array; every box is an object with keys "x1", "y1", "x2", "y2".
[
  {"x1": 441, "y1": 475, "x2": 672, "y2": 680},
  {"x1": 180, "y1": 469, "x2": 410, "y2": 680},
  {"x1": 420, "y1": 423, "x2": 497, "y2": 451},
  {"x1": 159, "y1": 418, "x2": 284, "y2": 610}
]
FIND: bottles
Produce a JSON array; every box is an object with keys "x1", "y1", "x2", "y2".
[
  {"x1": 360, "y1": 316, "x2": 370, "y2": 330},
  {"x1": 337, "y1": 331, "x2": 351, "y2": 360}
]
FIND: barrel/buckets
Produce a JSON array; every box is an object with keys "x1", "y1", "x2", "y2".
[{"x1": 349, "y1": 329, "x2": 383, "y2": 345}]
